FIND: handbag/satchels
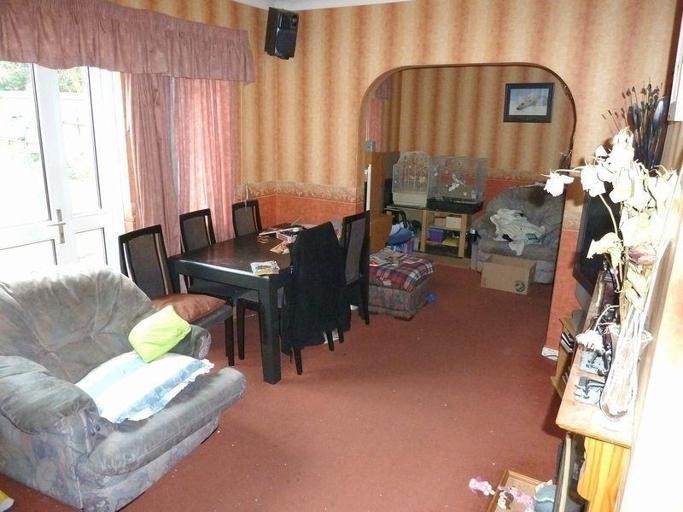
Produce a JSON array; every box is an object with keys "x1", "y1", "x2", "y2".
[{"x1": 386, "y1": 220, "x2": 417, "y2": 245}]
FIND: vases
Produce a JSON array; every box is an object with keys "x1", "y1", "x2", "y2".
[{"x1": 598, "y1": 325, "x2": 654, "y2": 416}]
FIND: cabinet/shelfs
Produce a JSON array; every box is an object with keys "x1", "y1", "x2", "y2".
[{"x1": 420, "y1": 210, "x2": 472, "y2": 259}]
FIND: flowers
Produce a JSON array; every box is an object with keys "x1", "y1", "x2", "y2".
[{"x1": 542, "y1": 125, "x2": 682, "y2": 405}]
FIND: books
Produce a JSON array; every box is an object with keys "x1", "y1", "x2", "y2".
[{"x1": 250, "y1": 260, "x2": 280, "y2": 275}]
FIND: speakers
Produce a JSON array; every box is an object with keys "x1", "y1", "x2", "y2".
[{"x1": 264, "y1": 7, "x2": 299, "y2": 60}]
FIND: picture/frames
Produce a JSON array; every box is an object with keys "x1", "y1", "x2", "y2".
[{"x1": 502, "y1": 83, "x2": 554, "y2": 122}]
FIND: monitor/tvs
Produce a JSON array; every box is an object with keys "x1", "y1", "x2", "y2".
[{"x1": 572, "y1": 180, "x2": 623, "y2": 298}]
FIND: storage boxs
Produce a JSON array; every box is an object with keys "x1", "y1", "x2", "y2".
[
  {"x1": 428, "y1": 216, "x2": 461, "y2": 242},
  {"x1": 479, "y1": 256, "x2": 536, "y2": 295}
]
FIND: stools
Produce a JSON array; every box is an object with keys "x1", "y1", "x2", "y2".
[{"x1": 367, "y1": 247, "x2": 433, "y2": 320}]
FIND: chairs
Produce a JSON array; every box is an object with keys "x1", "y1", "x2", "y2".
[
  {"x1": 468, "y1": 185, "x2": 565, "y2": 286},
  {"x1": 119, "y1": 197, "x2": 370, "y2": 376},
  {"x1": 0, "y1": 268, "x2": 246, "y2": 510}
]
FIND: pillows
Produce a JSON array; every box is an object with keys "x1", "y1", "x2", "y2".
[{"x1": 75, "y1": 304, "x2": 213, "y2": 427}]
